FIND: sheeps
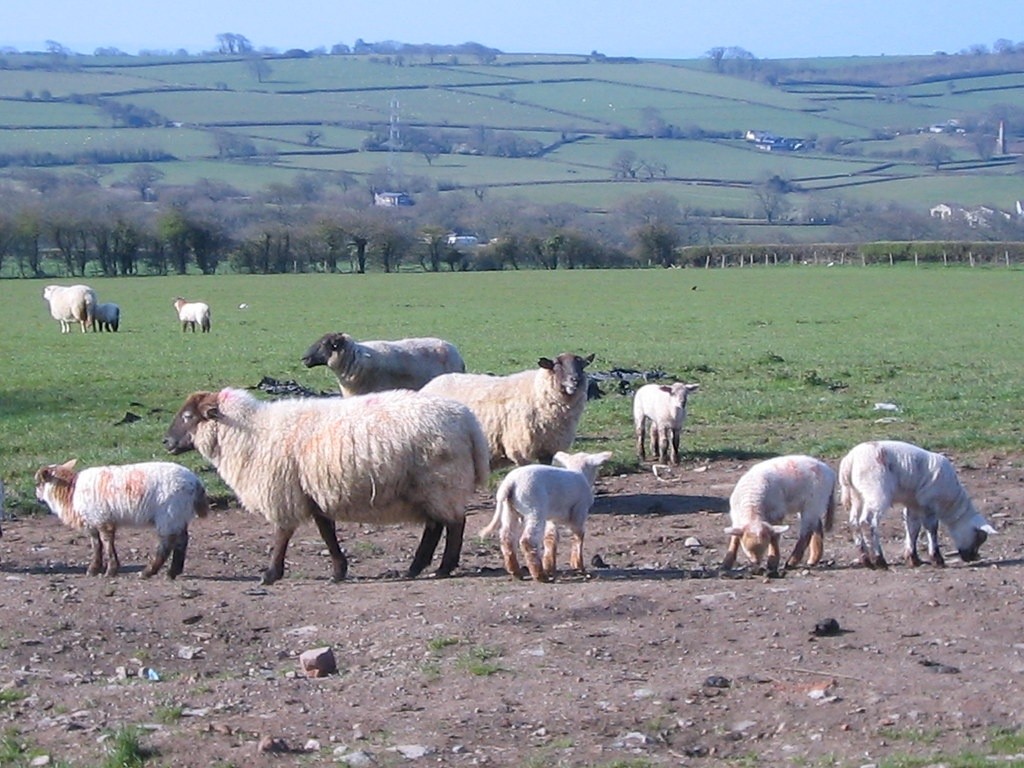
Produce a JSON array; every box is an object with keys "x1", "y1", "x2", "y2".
[
  {"x1": 633, "y1": 382, "x2": 701, "y2": 464},
  {"x1": 477, "y1": 450, "x2": 614, "y2": 584},
  {"x1": 162, "y1": 386, "x2": 489, "y2": 586},
  {"x1": 35, "y1": 459, "x2": 208, "y2": 581},
  {"x1": 300, "y1": 332, "x2": 466, "y2": 399},
  {"x1": 416, "y1": 352, "x2": 596, "y2": 471},
  {"x1": 839, "y1": 440, "x2": 998, "y2": 571},
  {"x1": 173, "y1": 298, "x2": 211, "y2": 333},
  {"x1": 717, "y1": 454, "x2": 837, "y2": 578},
  {"x1": 43, "y1": 284, "x2": 120, "y2": 334}
]
